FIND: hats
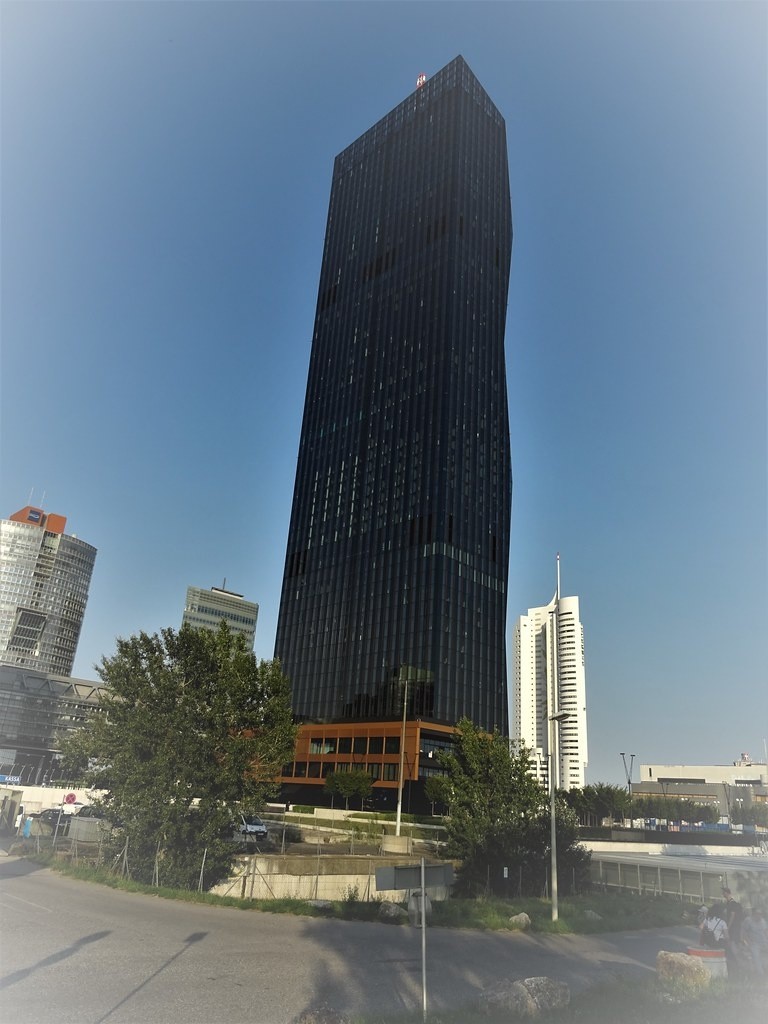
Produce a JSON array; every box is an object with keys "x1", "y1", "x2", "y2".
[{"x1": 720, "y1": 886, "x2": 731, "y2": 895}]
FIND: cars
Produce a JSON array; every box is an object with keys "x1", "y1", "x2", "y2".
[{"x1": 15, "y1": 801, "x2": 123, "y2": 836}]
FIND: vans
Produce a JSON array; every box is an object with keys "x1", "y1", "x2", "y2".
[{"x1": 225, "y1": 810, "x2": 268, "y2": 842}]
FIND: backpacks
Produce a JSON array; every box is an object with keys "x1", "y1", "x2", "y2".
[{"x1": 700, "y1": 918, "x2": 722, "y2": 950}]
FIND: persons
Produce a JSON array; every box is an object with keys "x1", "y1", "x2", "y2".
[{"x1": 696, "y1": 886, "x2": 768, "y2": 978}]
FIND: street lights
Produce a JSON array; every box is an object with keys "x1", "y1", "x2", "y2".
[
  {"x1": 658, "y1": 781, "x2": 671, "y2": 807},
  {"x1": 405, "y1": 751, "x2": 419, "y2": 813},
  {"x1": 537, "y1": 752, "x2": 550, "y2": 818},
  {"x1": 619, "y1": 752, "x2": 636, "y2": 830},
  {"x1": 721, "y1": 781, "x2": 732, "y2": 835}
]
